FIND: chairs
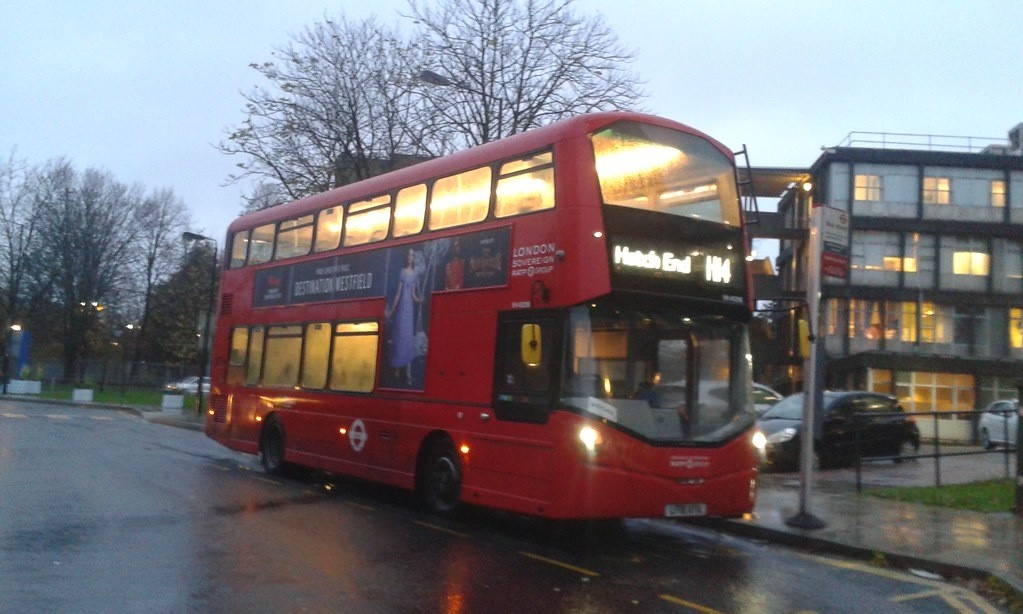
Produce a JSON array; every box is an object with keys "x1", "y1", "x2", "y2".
[{"x1": 578, "y1": 370, "x2": 604, "y2": 400}]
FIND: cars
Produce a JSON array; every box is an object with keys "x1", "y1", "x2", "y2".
[
  {"x1": 165, "y1": 376, "x2": 212, "y2": 396},
  {"x1": 754, "y1": 387, "x2": 923, "y2": 468},
  {"x1": 648, "y1": 378, "x2": 786, "y2": 438},
  {"x1": 978, "y1": 399, "x2": 1023, "y2": 452}
]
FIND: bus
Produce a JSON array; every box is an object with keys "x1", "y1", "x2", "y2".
[{"x1": 205, "y1": 110, "x2": 817, "y2": 531}]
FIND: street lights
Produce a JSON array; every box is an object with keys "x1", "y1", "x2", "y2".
[
  {"x1": 3, "y1": 324, "x2": 23, "y2": 395},
  {"x1": 182, "y1": 231, "x2": 218, "y2": 411},
  {"x1": 419, "y1": 69, "x2": 501, "y2": 136}
]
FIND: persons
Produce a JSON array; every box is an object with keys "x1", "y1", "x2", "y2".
[{"x1": 384, "y1": 239, "x2": 504, "y2": 387}]
